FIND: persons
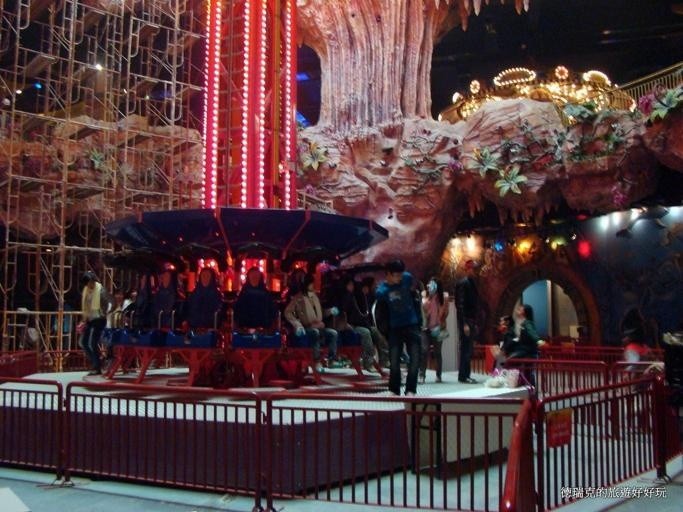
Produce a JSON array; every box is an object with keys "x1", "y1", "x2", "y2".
[
  {"x1": 499, "y1": 304, "x2": 546, "y2": 386},
  {"x1": 78, "y1": 270, "x2": 137, "y2": 375},
  {"x1": 454, "y1": 260, "x2": 481, "y2": 384},
  {"x1": 284, "y1": 258, "x2": 450, "y2": 398},
  {"x1": 618, "y1": 327, "x2": 656, "y2": 392}
]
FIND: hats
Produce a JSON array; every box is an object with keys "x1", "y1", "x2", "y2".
[{"x1": 384, "y1": 259, "x2": 406, "y2": 272}]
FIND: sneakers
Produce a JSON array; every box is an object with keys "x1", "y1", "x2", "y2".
[{"x1": 387, "y1": 375, "x2": 478, "y2": 398}]
